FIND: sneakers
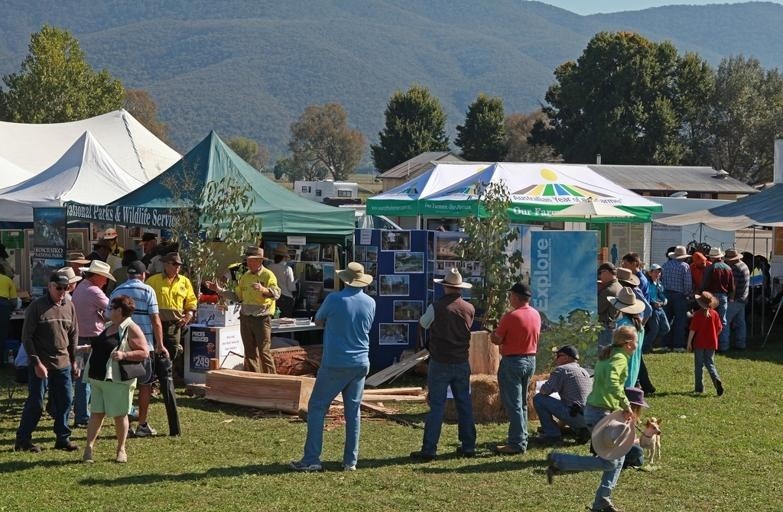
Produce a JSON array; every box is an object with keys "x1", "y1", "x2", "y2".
[
  {"x1": 409, "y1": 449, "x2": 437, "y2": 461},
  {"x1": 715, "y1": 379, "x2": 724, "y2": 396},
  {"x1": 689, "y1": 389, "x2": 705, "y2": 397},
  {"x1": 113, "y1": 446, "x2": 128, "y2": 463},
  {"x1": 457, "y1": 445, "x2": 477, "y2": 457},
  {"x1": 83, "y1": 448, "x2": 94, "y2": 461},
  {"x1": 341, "y1": 460, "x2": 356, "y2": 472},
  {"x1": 289, "y1": 459, "x2": 321, "y2": 473},
  {"x1": 137, "y1": 423, "x2": 156, "y2": 436},
  {"x1": 16, "y1": 438, "x2": 41, "y2": 454},
  {"x1": 496, "y1": 439, "x2": 526, "y2": 455},
  {"x1": 592, "y1": 496, "x2": 617, "y2": 512},
  {"x1": 56, "y1": 437, "x2": 77, "y2": 451},
  {"x1": 548, "y1": 451, "x2": 557, "y2": 486}
]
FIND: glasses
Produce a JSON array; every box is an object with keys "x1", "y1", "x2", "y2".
[{"x1": 557, "y1": 354, "x2": 565, "y2": 359}]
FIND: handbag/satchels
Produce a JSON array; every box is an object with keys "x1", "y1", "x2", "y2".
[{"x1": 118, "y1": 355, "x2": 148, "y2": 381}]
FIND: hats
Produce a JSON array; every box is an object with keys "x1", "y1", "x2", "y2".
[
  {"x1": 598, "y1": 262, "x2": 664, "y2": 315},
  {"x1": 335, "y1": 260, "x2": 374, "y2": 288},
  {"x1": 433, "y1": 267, "x2": 472, "y2": 290},
  {"x1": 590, "y1": 407, "x2": 639, "y2": 460},
  {"x1": 506, "y1": 282, "x2": 533, "y2": 297},
  {"x1": 622, "y1": 386, "x2": 651, "y2": 409},
  {"x1": 273, "y1": 247, "x2": 291, "y2": 258},
  {"x1": 667, "y1": 243, "x2": 743, "y2": 265},
  {"x1": 694, "y1": 289, "x2": 721, "y2": 310},
  {"x1": 244, "y1": 244, "x2": 268, "y2": 262},
  {"x1": 556, "y1": 345, "x2": 579, "y2": 360}
]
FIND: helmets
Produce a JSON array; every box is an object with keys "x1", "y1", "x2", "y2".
[{"x1": 48, "y1": 227, "x2": 184, "y2": 290}]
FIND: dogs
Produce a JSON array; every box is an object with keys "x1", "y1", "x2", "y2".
[{"x1": 639, "y1": 416, "x2": 662, "y2": 464}]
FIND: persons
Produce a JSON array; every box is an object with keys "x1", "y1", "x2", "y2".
[
  {"x1": 623, "y1": 385, "x2": 646, "y2": 465},
  {"x1": 531, "y1": 344, "x2": 595, "y2": 442},
  {"x1": 598, "y1": 240, "x2": 781, "y2": 397},
  {"x1": 407, "y1": 269, "x2": 477, "y2": 461},
  {"x1": 546, "y1": 327, "x2": 643, "y2": 509},
  {"x1": 288, "y1": 259, "x2": 377, "y2": 469},
  {"x1": 487, "y1": 283, "x2": 543, "y2": 454}
]
